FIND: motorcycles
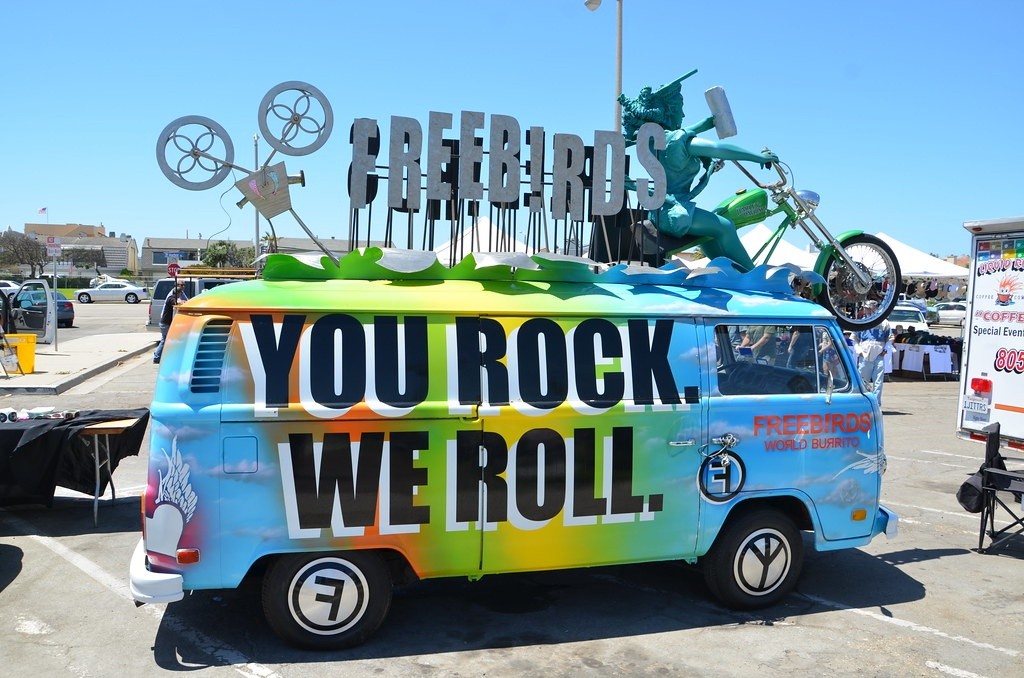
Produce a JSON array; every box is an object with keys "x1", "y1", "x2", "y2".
[{"x1": 586, "y1": 139, "x2": 904, "y2": 333}]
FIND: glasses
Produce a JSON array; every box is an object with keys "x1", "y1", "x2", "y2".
[{"x1": 177, "y1": 284, "x2": 185, "y2": 286}]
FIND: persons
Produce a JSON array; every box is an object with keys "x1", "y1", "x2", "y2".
[
  {"x1": 152, "y1": 280, "x2": 187, "y2": 363},
  {"x1": 716, "y1": 299, "x2": 895, "y2": 408},
  {"x1": 617, "y1": 67, "x2": 778, "y2": 274}
]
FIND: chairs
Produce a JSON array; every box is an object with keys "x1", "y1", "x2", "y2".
[{"x1": 956, "y1": 421, "x2": 1024, "y2": 553}]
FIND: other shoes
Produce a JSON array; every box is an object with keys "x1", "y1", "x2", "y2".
[{"x1": 153, "y1": 360, "x2": 160, "y2": 364}]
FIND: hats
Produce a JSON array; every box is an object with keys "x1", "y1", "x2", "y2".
[
  {"x1": 861, "y1": 300, "x2": 877, "y2": 309},
  {"x1": 872, "y1": 277, "x2": 967, "y2": 300}
]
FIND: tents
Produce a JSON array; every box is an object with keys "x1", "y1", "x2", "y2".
[
  {"x1": 689, "y1": 221, "x2": 839, "y2": 282},
  {"x1": 435, "y1": 216, "x2": 537, "y2": 265},
  {"x1": 828, "y1": 231, "x2": 973, "y2": 280}
]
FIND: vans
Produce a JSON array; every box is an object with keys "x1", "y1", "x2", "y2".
[
  {"x1": 123, "y1": 248, "x2": 903, "y2": 652},
  {"x1": 145, "y1": 277, "x2": 247, "y2": 335}
]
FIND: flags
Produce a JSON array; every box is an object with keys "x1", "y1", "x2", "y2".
[{"x1": 38, "y1": 207, "x2": 47, "y2": 214}]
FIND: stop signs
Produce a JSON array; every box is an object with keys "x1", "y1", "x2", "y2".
[{"x1": 167, "y1": 263, "x2": 180, "y2": 277}]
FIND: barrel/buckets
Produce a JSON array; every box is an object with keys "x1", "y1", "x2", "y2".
[{"x1": 3, "y1": 334, "x2": 38, "y2": 374}]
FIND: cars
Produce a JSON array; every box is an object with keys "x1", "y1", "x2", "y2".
[
  {"x1": 895, "y1": 298, "x2": 941, "y2": 328},
  {"x1": 879, "y1": 304, "x2": 929, "y2": 335},
  {"x1": 18, "y1": 291, "x2": 74, "y2": 327},
  {"x1": 75, "y1": 281, "x2": 149, "y2": 304},
  {"x1": 0, "y1": 280, "x2": 32, "y2": 309},
  {"x1": 0, "y1": 279, "x2": 55, "y2": 347},
  {"x1": 930, "y1": 301, "x2": 966, "y2": 327}
]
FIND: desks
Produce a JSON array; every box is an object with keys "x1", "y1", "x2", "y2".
[
  {"x1": 849, "y1": 343, "x2": 958, "y2": 382},
  {"x1": 0, "y1": 408, "x2": 150, "y2": 527}
]
